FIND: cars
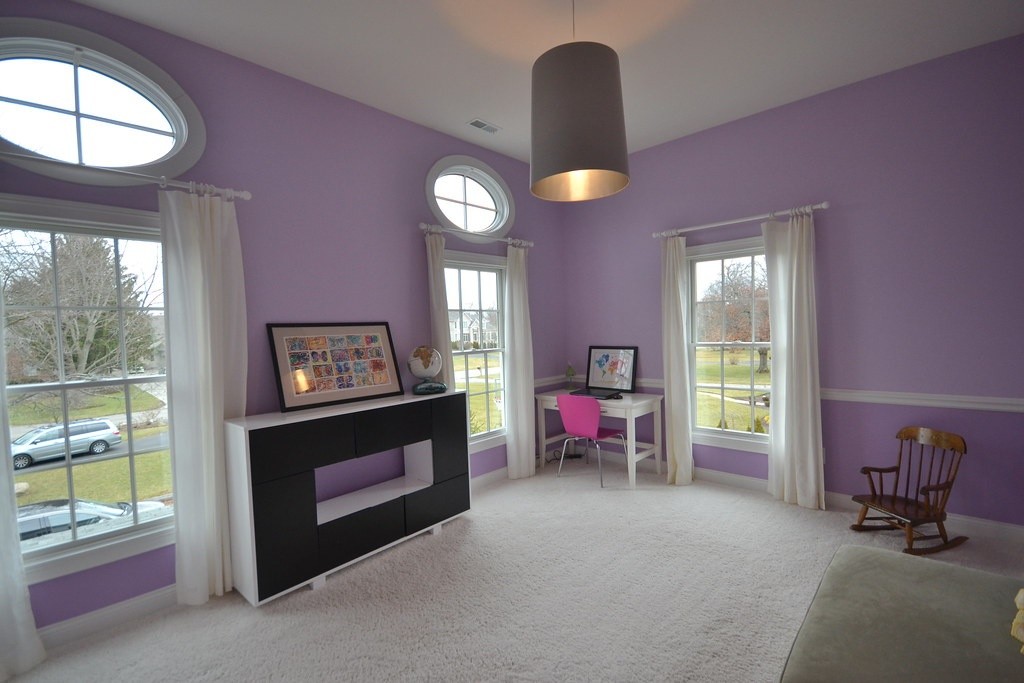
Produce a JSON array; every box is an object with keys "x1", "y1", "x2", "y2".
[{"x1": 16, "y1": 498, "x2": 172, "y2": 554}]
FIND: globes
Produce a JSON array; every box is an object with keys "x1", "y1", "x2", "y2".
[{"x1": 407, "y1": 345, "x2": 448, "y2": 395}]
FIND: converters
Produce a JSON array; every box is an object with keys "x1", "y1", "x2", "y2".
[{"x1": 560, "y1": 454, "x2": 582, "y2": 460}]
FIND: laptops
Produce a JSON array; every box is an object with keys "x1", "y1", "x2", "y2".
[{"x1": 569, "y1": 389, "x2": 620, "y2": 400}]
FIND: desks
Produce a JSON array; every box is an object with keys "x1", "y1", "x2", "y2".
[
  {"x1": 534, "y1": 386, "x2": 664, "y2": 486},
  {"x1": 779, "y1": 546, "x2": 1024, "y2": 683}
]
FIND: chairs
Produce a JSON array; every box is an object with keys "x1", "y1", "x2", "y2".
[
  {"x1": 849, "y1": 425, "x2": 970, "y2": 556},
  {"x1": 555, "y1": 393, "x2": 628, "y2": 489}
]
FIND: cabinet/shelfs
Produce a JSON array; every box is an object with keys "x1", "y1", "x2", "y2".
[{"x1": 223, "y1": 387, "x2": 474, "y2": 607}]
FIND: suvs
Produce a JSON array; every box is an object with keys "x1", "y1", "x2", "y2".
[{"x1": 10, "y1": 417, "x2": 122, "y2": 472}]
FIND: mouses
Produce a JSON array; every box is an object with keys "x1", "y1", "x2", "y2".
[{"x1": 613, "y1": 394, "x2": 622, "y2": 399}]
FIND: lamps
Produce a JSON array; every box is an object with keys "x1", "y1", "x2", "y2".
[{"x1": 528, "y1": 0, "x2": 630, "y2": 203}]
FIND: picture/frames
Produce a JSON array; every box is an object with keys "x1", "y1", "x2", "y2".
[
  {"x1": 266, "y1": 320, "x2": 406, "y2": 415},
  {"x1": 585, "y1": 344, "x2": 639, "y2": 394}
]
